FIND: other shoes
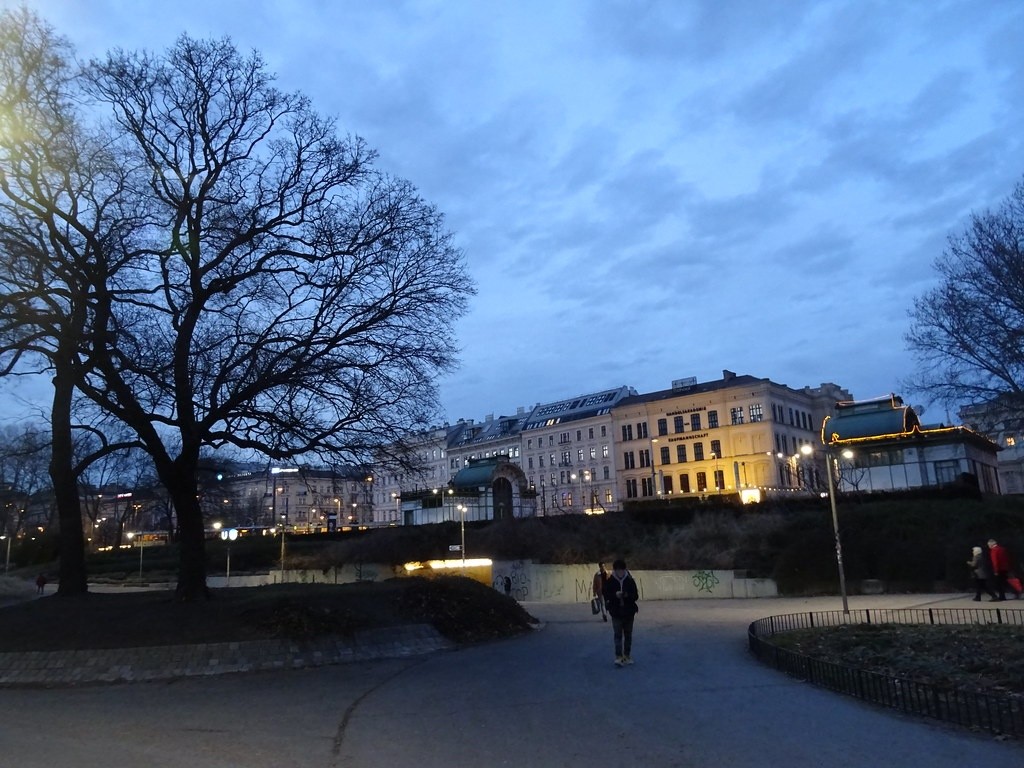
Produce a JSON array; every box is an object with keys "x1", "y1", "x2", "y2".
[
  {"x1": 624, "y1": 656, "x2": 634, "y2": 664},
  {"x1": 988, "y1": 596, "x2": 999, "y2": 602},
  {"x1": 603, "y1": 614, "x2": 607, "y2": 622},
  {"x1": 972, "y1": 597, "x2": 981, "y2": 601},
  {"x1": 997, "y1": 596, "x2": 1006, "y2": 601},
  {"x1": 614, "y1": 656, "x2": 624, "y2": 665}
]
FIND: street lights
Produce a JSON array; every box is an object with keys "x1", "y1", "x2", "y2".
[
  {"x1": 279, "y1": 511, "x2": 287, "y2": 564},
  {"x1": 448, "y1": 489, "x2": 454, "y2": 520},
  {"x1": 457, "y1": 505, "x2": 467, "y2": 563},
  {"x1": 709, "y1": 452, "x2": 722, "y2": 495},
  {"x1": 133, "y1": 504, "x2": 142, "y2": 526},
  {"x1": 334, "y1": 498, "x2": 340, "y2": 526},
  {"x1": 0, "y1": 534, "x2": 12, "y2": 574},
  {"x1": 432, "y1": 489, "x2": 439, "y2": 523},
  {"x1": 800, "y1": 442, "x2": 853, "y2": 615},
  {"x1": 570, "y1": 474, "x2": 591, "y2": 509},
  {"x1": 392, "y1": 492, "x2": 399, "y2": 522}
]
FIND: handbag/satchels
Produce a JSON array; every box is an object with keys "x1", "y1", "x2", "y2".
[
  {"x1": 1008, "y1": 572, "x2": 1022, "y2": 593},
  {"x1": 591, "y1": 594, "x2": 600, "y2": 615}
]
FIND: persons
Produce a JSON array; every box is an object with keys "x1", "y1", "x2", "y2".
[
  {"x1": 593, "y1": 559, "x2": 639, "y2": 665},
  {"x1": 966, "y1": 539, "x2": 1012, "y2": 603},
  {"x1": 36, "y1": 573, "x2": 45, "y2": 595}
]
